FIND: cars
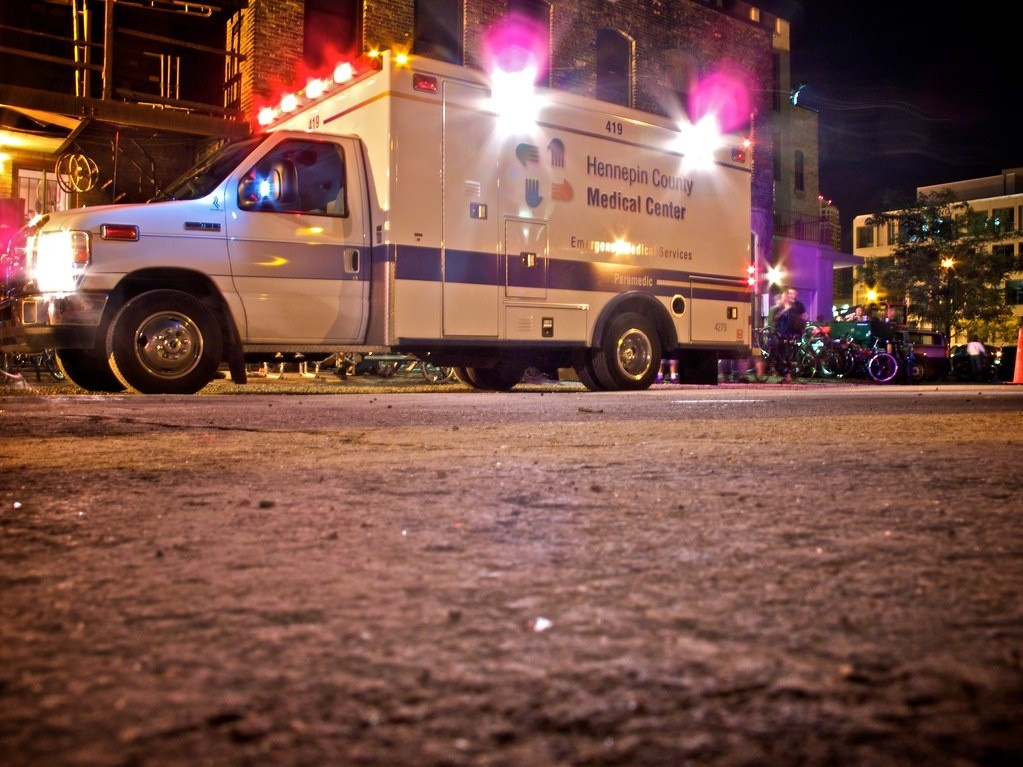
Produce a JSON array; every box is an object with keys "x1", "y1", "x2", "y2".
[{"x1": 949, "y1": 343, "x2": 1004, "y2": 383}]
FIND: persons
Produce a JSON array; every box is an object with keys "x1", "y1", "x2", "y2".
[
  {"x1": 717, "y1": 355, "x2": 763, "y2": 383},
  {"x1": 773, "y1": 288, "x2": 808, "y2": 384},
  {"x1": 834, "y1": 306, "x2": 907, "y2": 379},
  {"x1": 966, "y1": 336, "x2": 987, "y2": 379},
  {"x1": 655, "y1": 358, "x2": 680, "y2": 384}
]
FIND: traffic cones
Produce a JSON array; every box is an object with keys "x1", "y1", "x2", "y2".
[{"x1": 1007, "y1": 327, "x2": 1023, "y2": 384}]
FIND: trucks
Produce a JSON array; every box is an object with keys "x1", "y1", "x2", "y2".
[{"x1": 21, "y1": 42, "x2": 755, "y2": 397}]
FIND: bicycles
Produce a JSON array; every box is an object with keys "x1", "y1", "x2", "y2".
[
  {"x1": 718, "y1": 318, "x2": 924, "y2": 386},
  {"x1": 328, "y1": 349, "x2": 461, "y2": 386},
  {"x1": 0, "y1": 347, "x2": 64, "y2": 389}
]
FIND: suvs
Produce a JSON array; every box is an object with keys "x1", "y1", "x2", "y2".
[{"x1": 885, "y1": 326, "x2": 952, "y2": 385}]
